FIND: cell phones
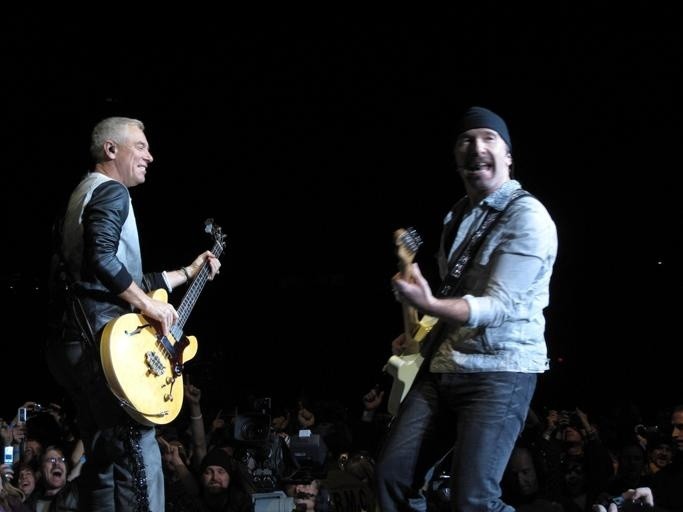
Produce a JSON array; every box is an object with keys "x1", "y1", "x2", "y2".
[
  {"x1": 3, "y1": 445, "x2": 14, "y2": 467},
  {"x1": 16, "y1": 406, "x2": 27, "y2": 426}
]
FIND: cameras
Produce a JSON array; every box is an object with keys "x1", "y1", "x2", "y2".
[
  {"x1": 637, "y1": 425, "x2": 659, "y2": 436},
  {"x1": 33, "y1": 403, "x2": 42, "y2": 412}
]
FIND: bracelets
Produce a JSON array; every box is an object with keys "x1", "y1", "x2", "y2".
[{"x1": 181, "y1": 266, "x2": 190, "y2": 283}]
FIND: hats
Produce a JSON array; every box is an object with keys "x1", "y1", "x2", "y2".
[
  {"x1": 449, "y1": 106, "x2": 511, "y2": 148},
  {"x1": 201, "y1": 448, "x2": 231, "y2": 475}
]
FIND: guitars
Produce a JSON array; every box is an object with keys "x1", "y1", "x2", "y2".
[
  {"x1": 100, "y1": 219, "x2": 226, "y2": 426},
  {"x1": 388, "y1": 227, "x2": 439, "y2": 419}
]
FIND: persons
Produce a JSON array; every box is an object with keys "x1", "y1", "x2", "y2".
[
  {"x1": 372, "y1": 105, "x2": 559, "y2": 511},
  {"x1": 53, "y1": 117, "x2": 221, "y2": 511},
  {"x1": 1, "y1": 380, "x2": 682, "y2": 511}
]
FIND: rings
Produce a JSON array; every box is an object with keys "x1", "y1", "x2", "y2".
[{"x1": 392, "y1": 288, "x2": 398, "y2": 292}]
